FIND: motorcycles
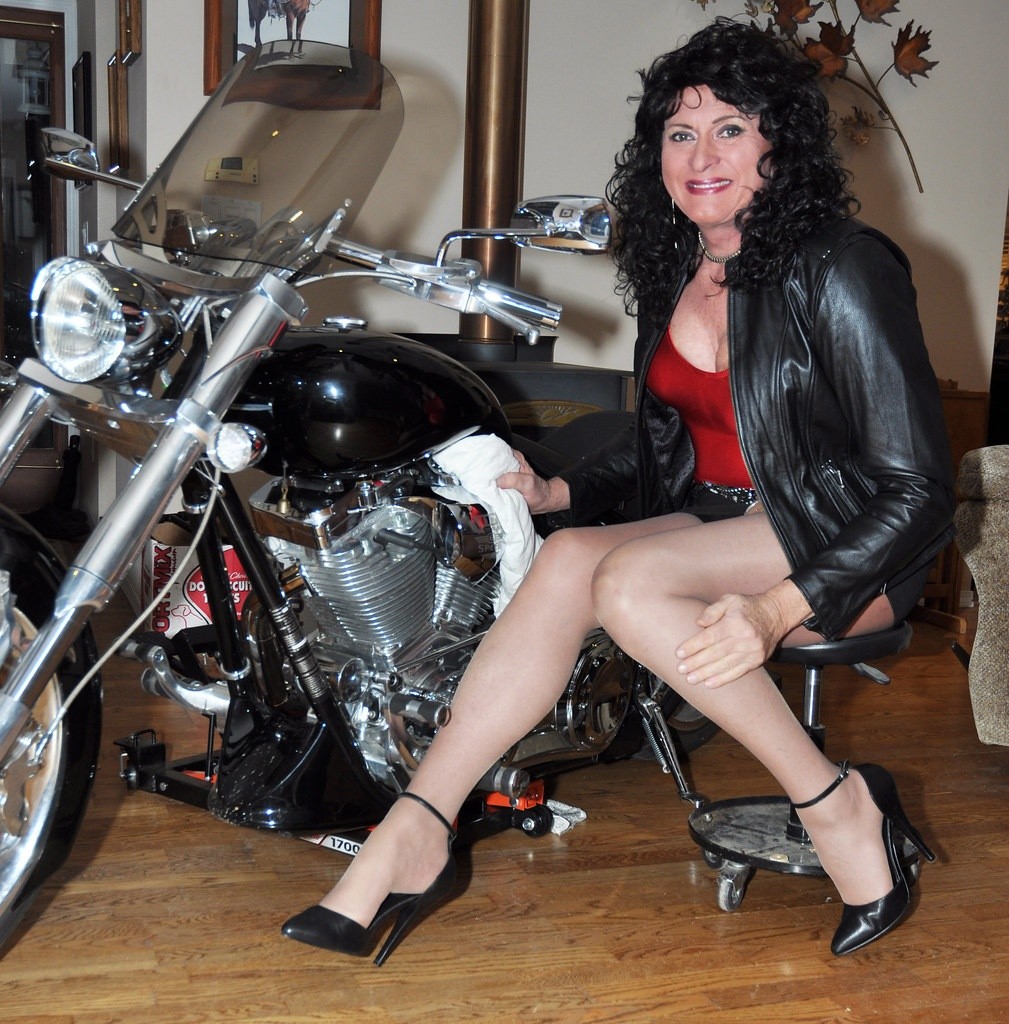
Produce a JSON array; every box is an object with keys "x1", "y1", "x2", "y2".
[{"x1": 0, "y1": 40, "x2": 722, "y2": 936}]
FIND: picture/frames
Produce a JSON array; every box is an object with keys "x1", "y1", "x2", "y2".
[
  {"x1": 72, "y1": 0, "x2": 144, "y2": 176},
  {"x1": 203, "y1": 0, "x2": 382, "y2": 111}
]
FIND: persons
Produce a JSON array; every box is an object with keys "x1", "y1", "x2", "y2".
[{"x1": 282, "y1": 22, "x2": 957, "y2": 966}]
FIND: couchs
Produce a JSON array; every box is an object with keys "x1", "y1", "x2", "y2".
[{"x1": 954, "y1": 444, "x2": 1009, "y2": 748}]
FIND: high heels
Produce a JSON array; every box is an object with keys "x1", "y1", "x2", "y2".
[
  {"x1": 281, "y1": 792, "x2": 458, "y2": 967},
  {"x1": 792, "y1": 759, "x2": 935, "y2": 957}
]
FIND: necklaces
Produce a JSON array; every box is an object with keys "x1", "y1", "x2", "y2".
[{"x1": 698, "y1": 232, "x2": 741, "y2": 263}]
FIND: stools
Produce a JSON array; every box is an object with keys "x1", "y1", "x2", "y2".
[{"x1": 634, "y1": 622, "x2": 935, "y2": 915}]
